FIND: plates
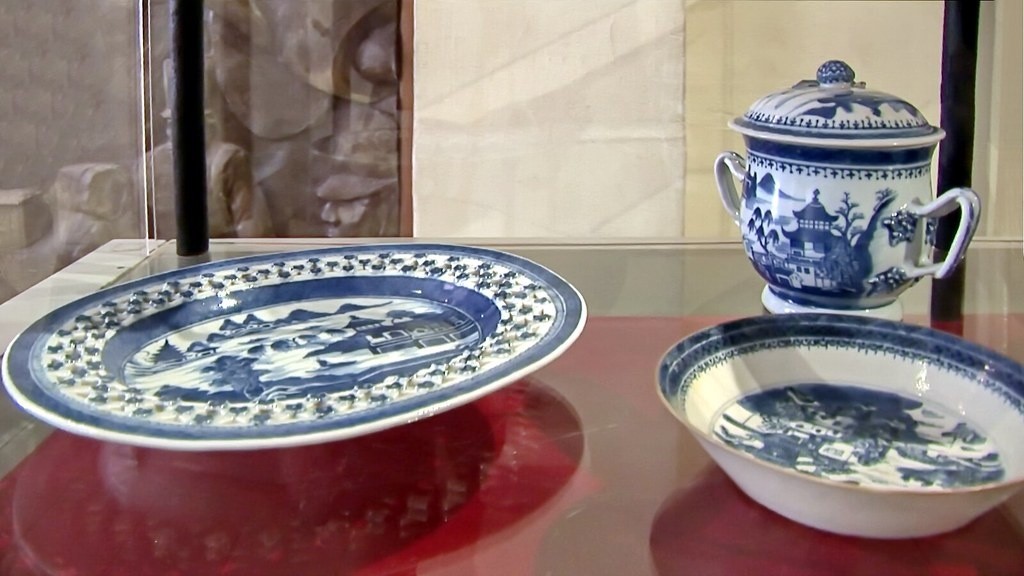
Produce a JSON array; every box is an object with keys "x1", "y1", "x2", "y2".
[{"x1": 0, "y1": 244, "x2": 589, "y2": 453}]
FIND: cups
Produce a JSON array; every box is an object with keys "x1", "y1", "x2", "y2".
[{"x1": 712, "y1": 60, "x2": 982, "y2": 322}]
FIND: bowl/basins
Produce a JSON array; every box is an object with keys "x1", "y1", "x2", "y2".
[{"x1": 654, "y1": 311, "x2": 1024, "y2": 541}]
